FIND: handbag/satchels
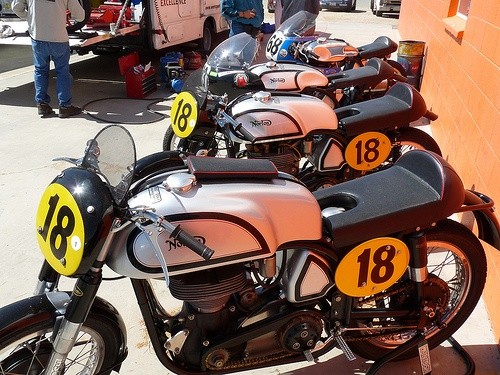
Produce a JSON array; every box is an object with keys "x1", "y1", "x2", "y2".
[{"x1": 1, "y1": 25, "x2": 16, "y2": 37}]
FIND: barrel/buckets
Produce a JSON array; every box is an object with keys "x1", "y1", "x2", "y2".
[{"x1": 398, "y1": 40, "x2": 426, "y2": 89}]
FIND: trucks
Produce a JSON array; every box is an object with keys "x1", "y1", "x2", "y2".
[{"x1": 69, "y1": 0, "x2": 231, "y2": 59}]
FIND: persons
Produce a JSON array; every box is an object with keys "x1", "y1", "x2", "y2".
[
  {"x1": 11, "y1": 0, "x2": 86, "y2": 118},
  {"x1": 281, "y1": 0, "x2": 320, "y2": 36},
  {"x1": 220, "y1": 0, "x2": 264, "y2": 37}
]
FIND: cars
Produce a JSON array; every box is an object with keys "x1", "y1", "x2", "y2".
[
  {"x1": 267, "y1": 0, "x2": 278, "y2": 13},
  {"x1": 319, "y1": 0, "x2": 357, "y2": 11},
  {"x1": 370, "y1": 0, "x2": 402, "y2": 17}
]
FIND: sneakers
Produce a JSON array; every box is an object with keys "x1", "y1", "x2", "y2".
[
  {"x1": 37, "y1": 102, "x2": 54, "y2": 115},
  {"x1": 58, "y1": 105, "x2": 82, "y2": 118}
]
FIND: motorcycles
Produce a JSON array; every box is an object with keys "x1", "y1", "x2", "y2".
[
  {"x1": 160, "y1": 10, "x2": 444, "y2": 192},
  {"x1": 0, "y1": 119, "x2": 500, "y2": 375}
]
